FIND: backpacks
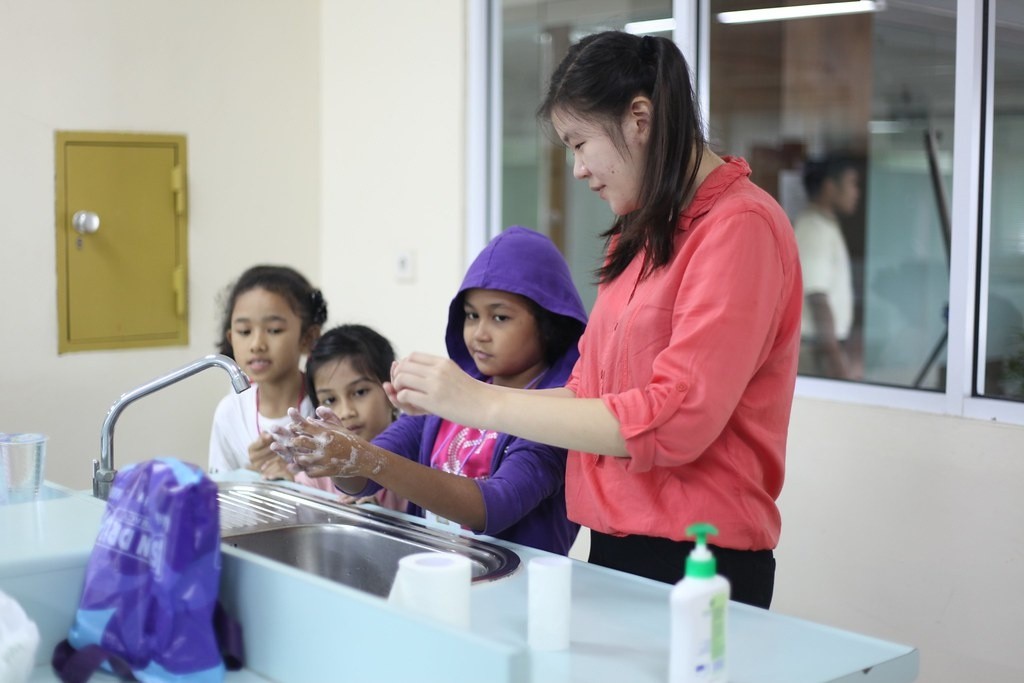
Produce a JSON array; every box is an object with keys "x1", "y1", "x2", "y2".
[{"x1": 52, "y1": 456, "x2": 241, "y2": 683}]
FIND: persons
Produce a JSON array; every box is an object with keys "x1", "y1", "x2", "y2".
[
  {"x1": 384, "y1": 31, "x2": 803, "y2": 608},
  {"x1": 208, "y1": 263, "x2": 329, "y2": 480},
  {"x1": 265, "y1": 223, "x2": 592, "y2": 558},
  {"x1": 294, "y1": 324, "x2": 425, "y2": 519},
  {"x1": 790, "y1": 153, "x2": 859, "y2": 382}
]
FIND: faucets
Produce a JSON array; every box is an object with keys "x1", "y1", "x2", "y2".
[{"x1": 91, "y1": 352, "x2": 255, "y2": 503}]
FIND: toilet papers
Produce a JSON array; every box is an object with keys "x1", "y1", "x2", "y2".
[
  {"x1": 527, "y1": 552, "x2": 575, "y2": 652},
  {"x1": 386, "y1": 550, "x2": 474, "y2": 631}
]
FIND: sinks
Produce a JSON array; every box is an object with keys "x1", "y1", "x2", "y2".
[{"x1": 220, "y1": 517, "x2": 522, "y2": 599}]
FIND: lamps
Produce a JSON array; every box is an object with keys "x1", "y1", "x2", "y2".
[{"x1": 570, "y1": 0, "x2": 888, "y2": 36}]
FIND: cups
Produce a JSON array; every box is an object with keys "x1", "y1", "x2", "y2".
[{"x1": 0, "y1": 433, "x2": 47, "y2": 505}]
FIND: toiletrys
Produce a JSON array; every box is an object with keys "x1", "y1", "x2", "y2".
[{"x1": 668, "y1": 521, "x2": 732, "y2": 683}]
